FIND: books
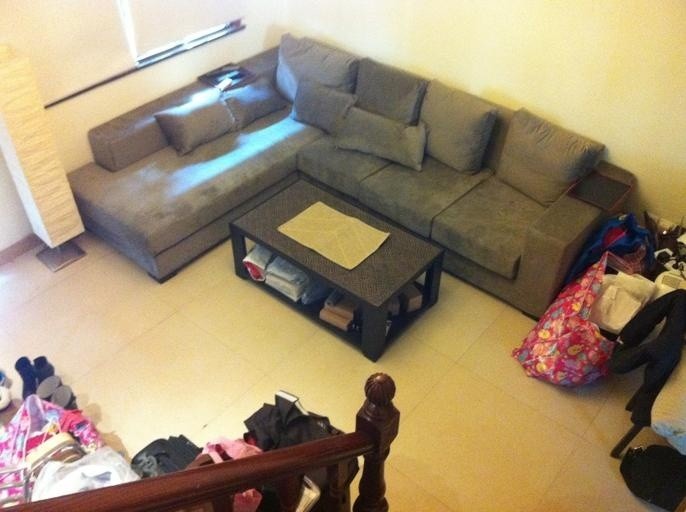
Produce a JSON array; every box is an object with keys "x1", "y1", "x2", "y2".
[
  {"x1": 264, "y1": 257, "x2": 311, "y2": 303},
  {"x1": 316, "y1": 288, "x2": 363, "y2": 332}
]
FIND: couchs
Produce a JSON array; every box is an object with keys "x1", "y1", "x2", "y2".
[{"x1": 66, "y1": 35, "x2": 638, "y2": 326}]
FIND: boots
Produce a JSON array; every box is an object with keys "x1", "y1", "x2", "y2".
[{"x1": 15, "y1": 356, "x2": 54, "y2": 398}]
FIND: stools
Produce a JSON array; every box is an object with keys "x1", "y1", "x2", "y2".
[{"x1": 612, "y1": 341, "x2": 686, "y2": 458}]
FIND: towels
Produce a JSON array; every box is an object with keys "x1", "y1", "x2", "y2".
[{"x1": 277, "y1": 202, "x2": 392, "y2": 269}]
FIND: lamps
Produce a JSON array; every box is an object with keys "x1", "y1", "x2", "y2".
[{"x1": 0, "y1": 44, "x2": 88, "y2": 272}]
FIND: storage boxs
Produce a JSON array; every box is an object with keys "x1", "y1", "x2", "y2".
[
  {"x1": 265, "y1": 270, "x2": 316, "y2": 302},
  {"x1": 318, "y1": 282, "x2": 423, "y2": 333}
]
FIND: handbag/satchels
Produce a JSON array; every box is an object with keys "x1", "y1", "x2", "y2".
[
  {"x1": 621, "y1": 445, "x2": 685, "y2": 510},
  {"x1": 510, "y1": 211, "x2": 686, "y2": 386}
]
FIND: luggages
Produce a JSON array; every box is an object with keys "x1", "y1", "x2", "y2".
[{"x1": 131, "y1": 434, "x2": 202, "y2": 480}]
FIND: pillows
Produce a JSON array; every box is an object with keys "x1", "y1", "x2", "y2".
[
  {"x1": 498, "y1": 107, "x2": 607, "y2": 207},
  {"x1": 290, "y1": 75, "x2": 357, "y2": 136},
  {"x1": 151, "y1": 88, "x2": 235, "y2": 156},
  {"x1": 273, "y1": 31, "x2": 358, "y2": 107},
  {"x1": 336, "y1": 105, "x2": 428, "y2": 171},
  {"x1": 415, "y1": 80, "x2": 500, "y2": 174},
  {"x1": 222, "y1": 76, "x2": 286, "y2": 130},
  {"x1": 355, "y1": 57, "x2": 430, "y2": 124}
]
